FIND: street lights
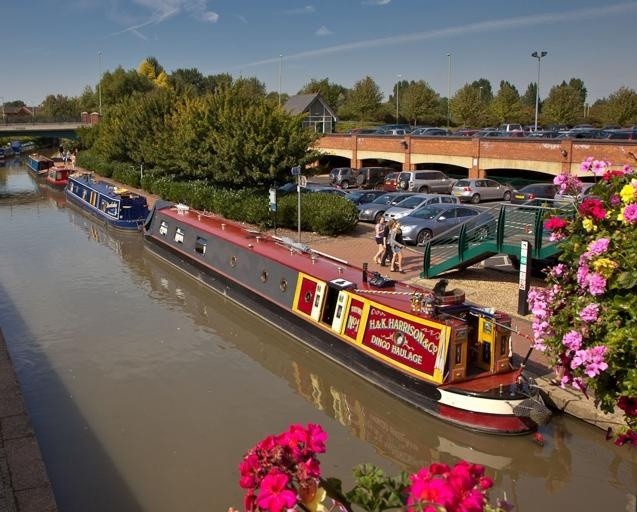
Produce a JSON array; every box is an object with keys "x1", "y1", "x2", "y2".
[
  {"x1": 97, "y1": 48, "x2": 106, "y2": 116},
  {"x1": 394, "y1": 71, "x2": 404, "y2": 124},
  {"x1": 444, "y1": 50, "x2": 452, "y2": 129},
  {"x1": 530, "y1": 49, "x2": 548, "y2": 132},
  {"x1": 274, "y1": 52, "x2": 284, "y2": 109}
]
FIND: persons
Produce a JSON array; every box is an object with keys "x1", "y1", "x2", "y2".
[
  {"x1": 60, "y1": 150, "x2": 76, "y2": 168},
  {"x1": 380, "y1": 219, "x2": 396, "y2": 268},
  {"x1": 390, "y1": 221, "x2": 405, "y2": 273},
  {"x1": 548, "y1": 366, "x2": 566, "y2": 385},
  {"x1": 373, "y1": 216, "x2": 385, "y2": 264}
]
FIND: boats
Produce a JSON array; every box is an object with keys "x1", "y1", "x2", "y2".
[
  {"x1": 22, "y1": 151, "x2": 56, "y2": 178},
  {"x1": 44, "y1": 163, "x2": 80, "y2": 191},
  {"x1": 61, "y1": 169, "x2": 152, "y2": 233},
  {"x1": 137, "y1": 197, "x2": 554, "y2": 439}
]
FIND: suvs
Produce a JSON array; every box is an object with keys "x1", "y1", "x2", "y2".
[
  {"x1": 328, "y1": 166, "x2": 362, "y2": 190},
  {"x1": 396, "y1": 170, "x2": 460, "y2": 194},
  {"x1": 355, "y1": 166, "x2": 398, "y2": 192}
]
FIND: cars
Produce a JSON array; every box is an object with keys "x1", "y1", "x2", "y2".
[
  {"x1": 553, "y1": 180, "x2": 599, "y2": 211},
  {"x1": 381, "y1": 202, "x2": 497, "y2": 247},
  {"x1": 381, "y1": 193, "x2": 462, "y2": 223},
  {"x1": 345, "y1": 190, "x2": 389, "y2": 207},
  {"x1": 301, "y1": 185, "x2": 353, "y2": 201},
  {"x1": 355, "y1": 191, "x2": 424, "y2": 225},
  {"x1": 451, "y1": 176, "x2": 518, "y2": 204},
  {"x1": 373, "y1": 120, "x2": 636, "y2": 141},
  {"x1": 509, "y1": 183, "x2": 565, "y2": 205},
  {"x1": 383, "y1": 168, "x2": 402, "y2": 193}
]
